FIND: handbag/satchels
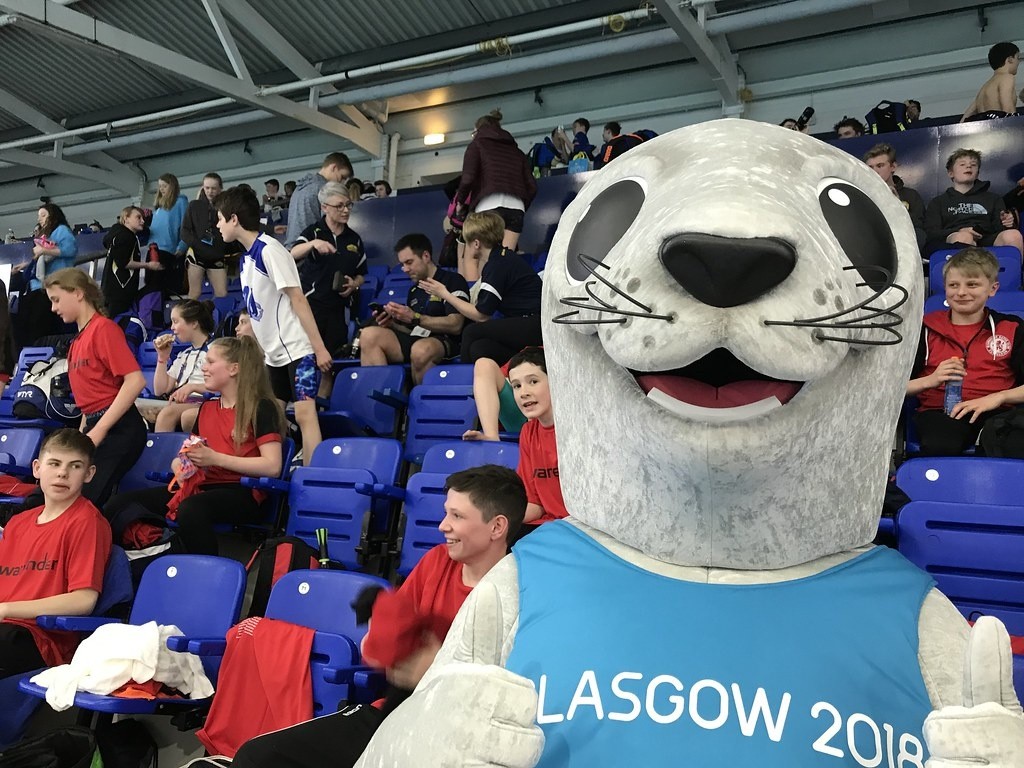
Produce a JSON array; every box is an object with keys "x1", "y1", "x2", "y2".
[{"x1": 567, "y1": 151, "x2": 588, "y2": 174}]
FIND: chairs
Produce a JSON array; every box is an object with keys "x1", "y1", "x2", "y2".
[{"x1": 1, "y1": 230, "x2": 1023, "y2": 760}]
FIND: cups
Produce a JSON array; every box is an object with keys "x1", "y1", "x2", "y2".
[{"x1": 328, "y1": 270, "x2": 348, "y2": 292}]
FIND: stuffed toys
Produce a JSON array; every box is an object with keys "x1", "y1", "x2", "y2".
[{"x1": 355, "y1": 118, "x2": 1024, "y2": 768}]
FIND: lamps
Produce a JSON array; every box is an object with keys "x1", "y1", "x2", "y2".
[{"x1": 423, "y1": 134, "x2": 445, "y2": 145}]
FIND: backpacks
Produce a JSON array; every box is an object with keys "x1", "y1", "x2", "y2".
[
  {"x1": 247, "y1": 536, "x2": 321, "y2": 619},
  {"x1": 527, "y1": 136, "x2": 561, "y2": 167},
  {"x1": 865, "y1": 100, "x2": 907, "y2": 135},
  {"x1": 12, "y1": 357, "x2": 82, "y2": 428}
]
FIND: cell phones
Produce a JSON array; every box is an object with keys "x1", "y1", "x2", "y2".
[{"x1": 368, "y1": 302, "x2": 392, "y2": 320}]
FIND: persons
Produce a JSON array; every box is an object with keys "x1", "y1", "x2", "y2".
[
  {"x1": 905, "y1": 247, "x2": 1024, "y2": 462},
  {"x1": 864, "y1": 142, "x2": 927, "y2": 250},
  {"x1": 923, "y1": 148, "x2": 1024, "y2": 267},
  {"x1": 779, "y1": 119, "x2": 809, "y2": 134},
  {"x1": 834, "y1": 117, "x2": 865, "y2": 139},
  {"x1": 975, "y1": 41, "x2": 1020, "y2": 119},
  {"x1": 0, "y1": 108, "x2": 621, "y2": 556},
  {"x1": 0, "y1": 427, "x2": 112, "y2": 680},
  {"x1": 227, "y1": 463, "x2": 528, "y2": 768}
]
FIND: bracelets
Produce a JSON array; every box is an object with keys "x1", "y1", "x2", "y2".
[{"x1": 412, "y1": 312, "x2": 421, "y2": 324}]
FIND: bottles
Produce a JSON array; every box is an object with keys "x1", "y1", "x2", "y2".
[
  {"x1": 944, "y1": 356, "x2": 963, "y2": 416},
  {"x1": 148, "y1": 242, "x2": 159, "y2": 261},
  {"x1": 4, "y1": 228, "x2": 12, "y2": 244},
  {"x1": 54, "y1": 376, "x2": 61, "y2": 386}
]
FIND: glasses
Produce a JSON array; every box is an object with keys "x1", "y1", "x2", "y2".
[
  {"x1": 471, "y1": 129, "x2": 481, "y2": 138},
  {"x1": 324, "y1": 202, "x2": 354, "y2": 212}
]
